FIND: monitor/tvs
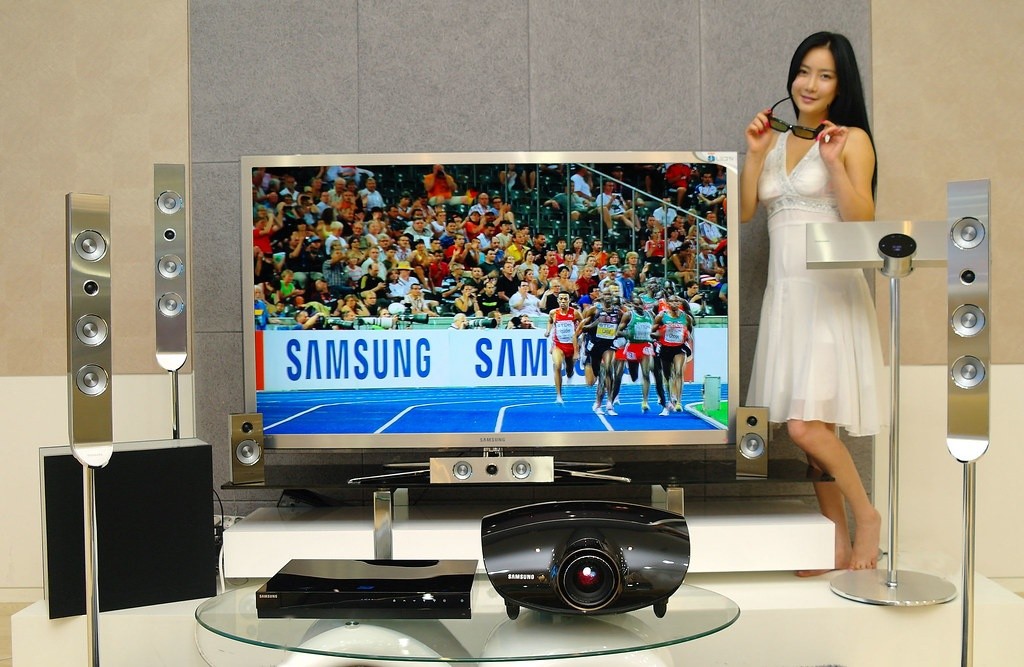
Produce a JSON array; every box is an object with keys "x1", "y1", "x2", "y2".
[{"x1": 240, "y1": 155, "x2": 739, "y2": 483}]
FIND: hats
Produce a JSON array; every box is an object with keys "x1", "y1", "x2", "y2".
[
  {"x1": 607, "y1": 265, "x2": 618, "y2": 271},
  {"x1": 308, "y1": 235, "x2": 321, "y2": 242},
  {"x1": 396, "y1": 261, "x2": 415, "y2": 270},
  {"x1": 501, "y1": 220, "x2": 512, "y2": 224}
]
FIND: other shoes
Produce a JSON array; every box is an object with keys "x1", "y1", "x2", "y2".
[
  {"x1": 671, "y1": 396, "x2": 677, "y2": 412},
  {"x1": 659, "y1": 407, "x2": 669, "y2": 416},
  {"x1": 566, "y1": 375, "x2": 572, "y2": 384},
  {"x1": 613, "y1": 397, "x2": 620, "y2": 405},
  {"x1": 592, "y1": 403, "x2": 604, "y2": 414},
  {"x1": 641, "y1": 402, "x2": 649, "y2": 411},
  {"x1": 607, "y1": 410, "x2": 618, "y2": 415},
  {"x1": 676, "y1": 403, "x2": 683, "y2": 413},
  {"x1": 556, "y1": 396, "x2": 563, "y2": 405},
  {"x1": 606, "y1": 402, "x2": 613, "y2": 410},
  {"x1": 669, "y1": 403, "x2": 674, "y2": 412}
]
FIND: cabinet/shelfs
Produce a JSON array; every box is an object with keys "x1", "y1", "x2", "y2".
[{"x1": 221, "y1": 458, "x2": 835, "y2": 581}]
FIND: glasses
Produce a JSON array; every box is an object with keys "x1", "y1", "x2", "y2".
[{"x1": 767, "y1": 96, "x2": 830, "y2": 140}]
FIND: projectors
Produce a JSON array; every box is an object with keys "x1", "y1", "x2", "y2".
[{"x1": 481, "y1": 499, "x2": 689, "y2": 620}]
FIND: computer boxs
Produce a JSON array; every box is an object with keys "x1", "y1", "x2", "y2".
[{"x1": 39, "y1": 435, "x2": 217, "y2": 621}]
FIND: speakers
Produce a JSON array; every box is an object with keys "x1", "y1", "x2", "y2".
[
  {"x1": 153, "y1": 163, "x2": 187, "y2": 372},
  {"x1": 230, "y1": 412, "x2": 265, "y2": 485},
  {"x1": 947, "y1": 179, "x2": 989, "y2": 463},
  {"x1": 430, "y1": 457, "x2": 554, "y2": 483},
  {"x1": 66, "y1": 192, "x2": 113, "y2": 466},
  {"x1": 736, "y1": 406, "x2": 768, "y2": 480}
]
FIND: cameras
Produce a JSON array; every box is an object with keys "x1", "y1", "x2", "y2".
[
  {"x1": 699, "y1": 292, "x2": 707, "y2": 301},
  {"x1": 464, "y1": 318, "x2": 497, "y2": 329},
  {"x1": 319, "y1": 315, "x2": 354, "y2": 330},
  {"x1": 615, "y1": 195, "x2": 620, "y2": 200},
  {"x1": 395, "y1": 314, "x2": 429, "y2": 324}
]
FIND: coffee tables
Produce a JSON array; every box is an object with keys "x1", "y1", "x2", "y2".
[{"x1": 194, "y1": 575, "x2": 741, "y2": 667}]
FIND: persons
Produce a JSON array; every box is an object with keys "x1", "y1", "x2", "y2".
[
  {"x1": 253, "y1": 163, "x2": 727, "y2": 415},
  {"x1": 739, "y1": 30, "x2": 885, "y2": 577}
]
